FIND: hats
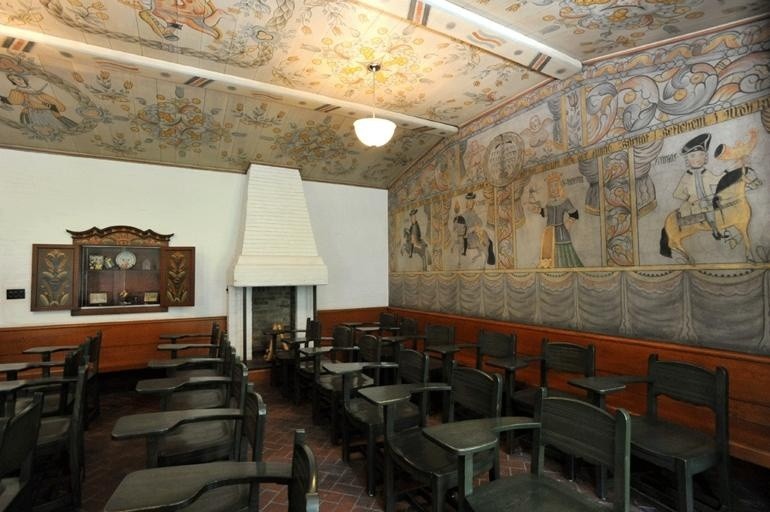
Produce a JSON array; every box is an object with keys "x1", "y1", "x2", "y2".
[
  {"x1": 409, "y1": 209, "x2": 417, "y2": 216},
  {"x1": 466, "y1": 192, "x2": 476, "y2": 199},
  {"x1": 681, "y1": 134, "x2": 711, "y2": 155}
]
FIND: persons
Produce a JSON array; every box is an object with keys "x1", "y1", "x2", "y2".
[
  {"x1": 674, "y1": 135, "x2": 729, "y2": 240},
  {"x1": 530, "y1": 174, "x2": 584, "y2": 269},
  {"x1": 458, "y1": 192, "x2": 483, "y2": 240},
  {"x1": 409, "y1": 209, "x2": 421, "y2": 258}
]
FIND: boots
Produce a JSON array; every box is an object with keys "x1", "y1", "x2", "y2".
[
  {"x1": 461, "y1": 238, "x2": 467, "y2": 256},
  {"x1": 409, "y1": 243, "x2": 414, "y2": 257}
]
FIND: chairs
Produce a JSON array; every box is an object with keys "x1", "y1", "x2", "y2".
[
  {"x1": 0, "y1": 327, "x2": 103, "y2": 511},
  {"x1": 459, "y1": 386, "x2": 631, "y2": 511},
  {"x1": 264, "y1": 313, "x2": 596, "y2": 511},
  {"x1": 111, "y1": 319, "x2": 268, "y2": 510}
]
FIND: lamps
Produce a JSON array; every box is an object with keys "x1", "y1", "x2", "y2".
[{"x1": 353, "y1": 64, "x2": 396, "y2": 147}]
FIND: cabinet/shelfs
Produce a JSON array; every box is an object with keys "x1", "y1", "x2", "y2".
[{"x1": 80, "y1": 244, "x2": 161, "y2": 309}]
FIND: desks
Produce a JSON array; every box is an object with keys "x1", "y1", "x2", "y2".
[
  {"x1": 630, "y1": 353, "x2": 728, "y2": 512},
  {"x1": 103, "y1": 460, "x2": 291, "y2": 511},
  {"x1": 568, "y1": 375, "x2": 646, "y2": 394}
]
